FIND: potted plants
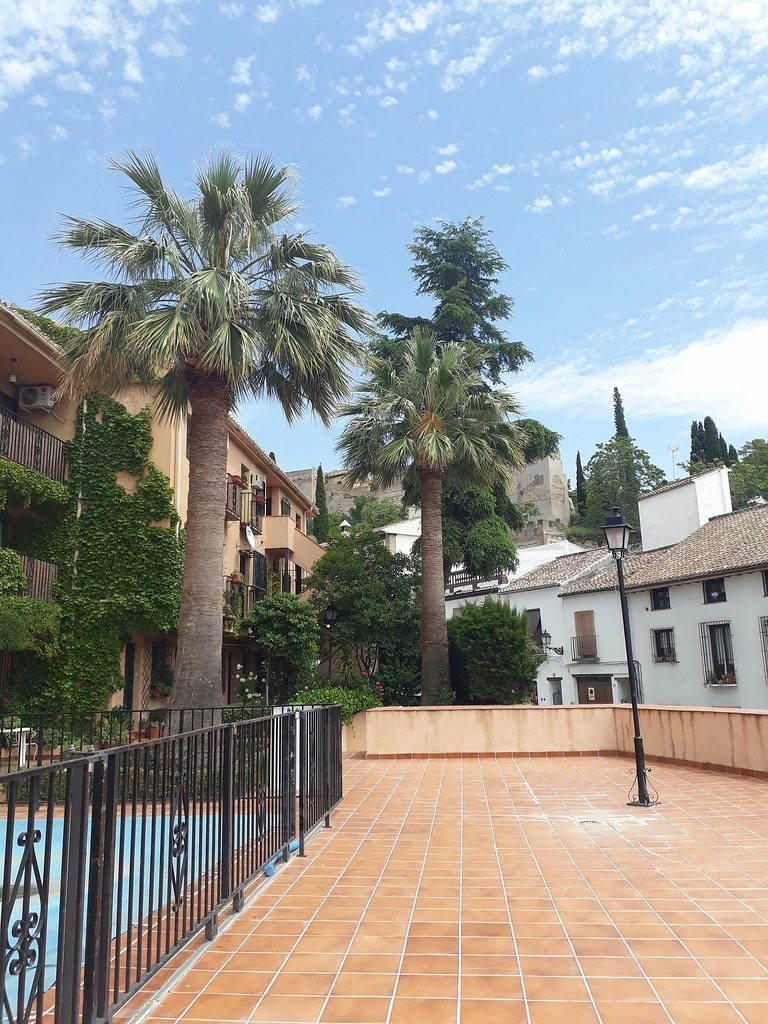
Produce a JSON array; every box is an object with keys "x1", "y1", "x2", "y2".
[
  {"x1": 233, "y1": 475, "x2": 242, "y2": 484},
  {"x1": 98, "y1": 706, "x2": 167, "y2": 750},
  {"x1": 240, "y1": 474, "x2": 249, "y2": 490},
  {"x1": 223, "y1": 604, "x2": 236, "y2": 634},
  {"x1": 233, "y1": 568, "x2": 248, "y2": 596},
  {"x1": 257, "y1": 492, "x2": 266, "y2": 505}
]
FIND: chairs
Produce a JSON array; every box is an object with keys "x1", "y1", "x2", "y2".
[{"x1": 2, "y1": 716, "x2": 37, "y2": 764}]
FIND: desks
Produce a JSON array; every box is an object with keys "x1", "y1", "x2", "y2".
[{"x1": 0, "y1": 728, "x2": 32, "y2": 766}]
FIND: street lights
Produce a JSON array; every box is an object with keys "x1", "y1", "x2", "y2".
[
  {"x1": 599, "y1": 506, "x2": 659, "y2": 807},
  {"x1": 325, "y1": 600, "x2": 339, "y2": 684}
]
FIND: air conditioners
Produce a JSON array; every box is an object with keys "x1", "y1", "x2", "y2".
[
  {"x1": 19, "y1": 385, "x2": 55, "y2": 409},
  {"x1": 250, "y1": 473, "x2": 263, "y2": 489}
]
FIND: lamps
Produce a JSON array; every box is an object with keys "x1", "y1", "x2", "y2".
[
  {"x1": 9, "y1": 357, "x2": 17, "y2": 383},
  {"x1": 539, "y1": 630, "x2": 563, "y2": 655}
]
